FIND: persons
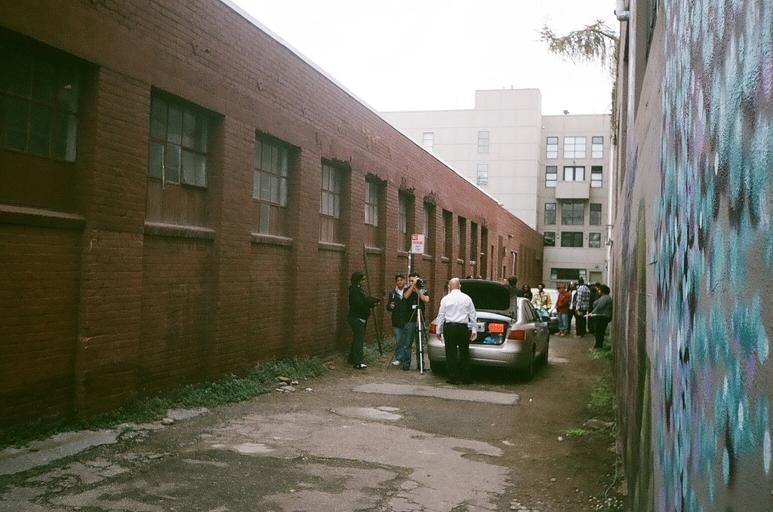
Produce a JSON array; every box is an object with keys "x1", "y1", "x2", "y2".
[
  {"x1": 399, "y1": 271, "x2": 430, "y2": 373},
  {"x1": 385, "y1": 273, "x2": 409, "y2": 366},
  {"x1": 434, "y1": 277, "x2": 478, "y2": 385},
  {"x1": 587, "y1": 284, "x2": 612, "y2": 353},
  {"x1": 345, "y1": 271, "x2": 381, "y2": 369},
  {"x1": 465, "y1": 274, "x2": 601, "y2": 336}
]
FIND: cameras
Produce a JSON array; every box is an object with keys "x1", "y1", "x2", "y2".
[{"x1": 415, "y1": 278, "x2": 424, "y2": 289}]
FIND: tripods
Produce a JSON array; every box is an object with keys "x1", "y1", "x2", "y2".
[{"x1": 387, "y1": 305, "x2": 430, "y2": 375}]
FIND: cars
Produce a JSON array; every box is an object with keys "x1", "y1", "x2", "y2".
[
  {"x1": 529, "y1": 288, "x2": 561, "y2": 333},
  {"x1": 427, "y1": 279, "x2": 551, "y2": 381}
]
